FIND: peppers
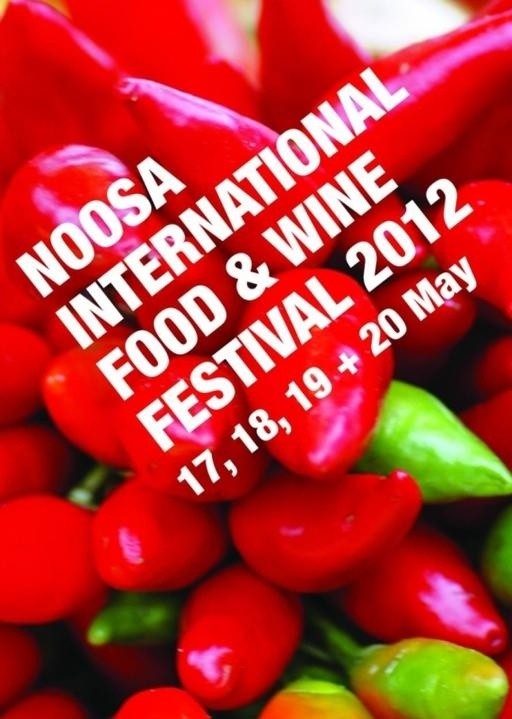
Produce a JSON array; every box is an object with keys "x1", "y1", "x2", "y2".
[{"x1": 0, "y1": 1, "x2": 512, "y2": 717}]
